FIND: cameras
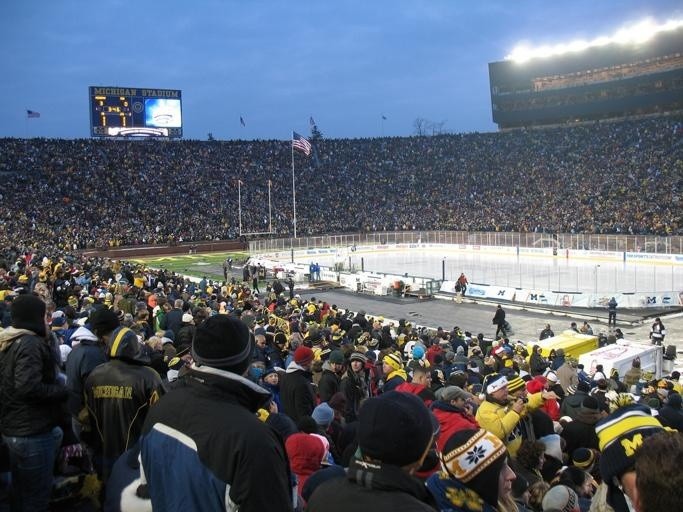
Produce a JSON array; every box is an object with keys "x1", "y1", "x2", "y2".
[{"x1": 515, "y1": 396, "x2": 528, "y2": 403}]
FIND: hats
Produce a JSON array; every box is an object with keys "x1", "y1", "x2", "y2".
[{"x1": 11, "y1": 271, "x2": 683, "y2": 512}]
